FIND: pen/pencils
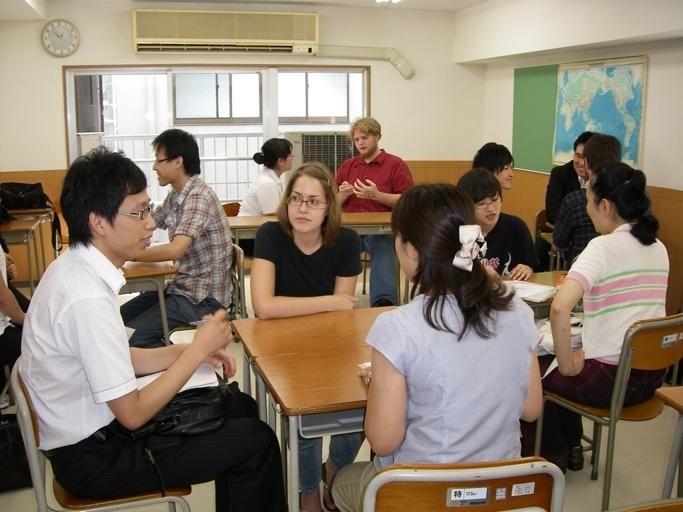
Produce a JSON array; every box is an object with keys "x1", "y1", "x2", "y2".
[{"x1": 189, "y1": 320, "x2": 208, "y2": 326}]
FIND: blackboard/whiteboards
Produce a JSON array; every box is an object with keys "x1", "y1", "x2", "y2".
[{"x1": 511, "y1": 64, "x2": 559, "y2": 175}]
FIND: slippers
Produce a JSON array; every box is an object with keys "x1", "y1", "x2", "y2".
[{"x1": 321, "y1": 463, "x2": 340, "y2": 512}]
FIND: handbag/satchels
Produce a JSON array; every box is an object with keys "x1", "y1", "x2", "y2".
[
  {"x1": 108, "y1": 372, "x2": 233, "y2": 439},
  {"x1": 0, "y1": 183, "x2": 45, "y2": 210}
]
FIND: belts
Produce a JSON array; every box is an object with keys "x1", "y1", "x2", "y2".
[{"x1": 40, "y1": 428, "x2": 107, "y2": 458}]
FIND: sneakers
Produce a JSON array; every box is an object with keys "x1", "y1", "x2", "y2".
[
  {"x1": 520, "y1": 437, "x2": 584, "y2": 473},
  {"x1": 377, "y1": 299, "x2": 393, "y2": 307}
]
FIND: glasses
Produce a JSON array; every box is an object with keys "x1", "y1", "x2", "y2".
[
  {"x1": 155, "y1": 157, "x2": 169, "y2": 165},
  {"x1": 117, "y1": 203, "x2": 154, "y2": 220},
  {"x1": 285, "y1": 197, "x2": 328, "y2": 209},
  {"x1": 477, "y1": 194, "x2": 503, "y2": 209}
]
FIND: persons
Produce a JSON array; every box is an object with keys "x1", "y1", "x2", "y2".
[
  {"x1": 15, "y1": 147, "x2": 288, "y2": 510},
  {"x1": 0, "y1": 233, "x2": 33, "y2": 409},
  {"x1": 473, "y1": 142, "x2": 515, "y2": 189},
  {"x1": 233, "y1": 136, "x2": 296, "y2": 218},
  {"x1": 330, "y1": 118, "x2": 419, "y2": 308},
  {"x1": 246, "y1": 160, "x2": 367, "y2": 511},
  {"x1": 333, "y1": 179, "x2": 548, "y2": 511},
  {"x1": 123, "y1": 129, "x2": 240, "y2": 347},
  {"x1": 538, "y1": 132, "x2": 611, "y2": 273},
  {"x1": 519, "y1": 160, "x2": 671, "y2": 474},
  {"x1": 455, "y1": 171, "x2": 540, "y2": 282},
  {"x1": 550, "y1": 133, "x2": 627, "y2": 267}
]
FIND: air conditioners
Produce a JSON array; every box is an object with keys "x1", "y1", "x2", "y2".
[
  {"x1": 287, "y1": 131, "x2": 359, "y2": 176},
  {"x1": 130, "y1": 10, "x2": 319, "y2": 56}
]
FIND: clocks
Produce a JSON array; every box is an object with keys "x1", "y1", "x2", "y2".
[{"x1": 39, "y1": 19, "x2": 81, "y2": 56}]
